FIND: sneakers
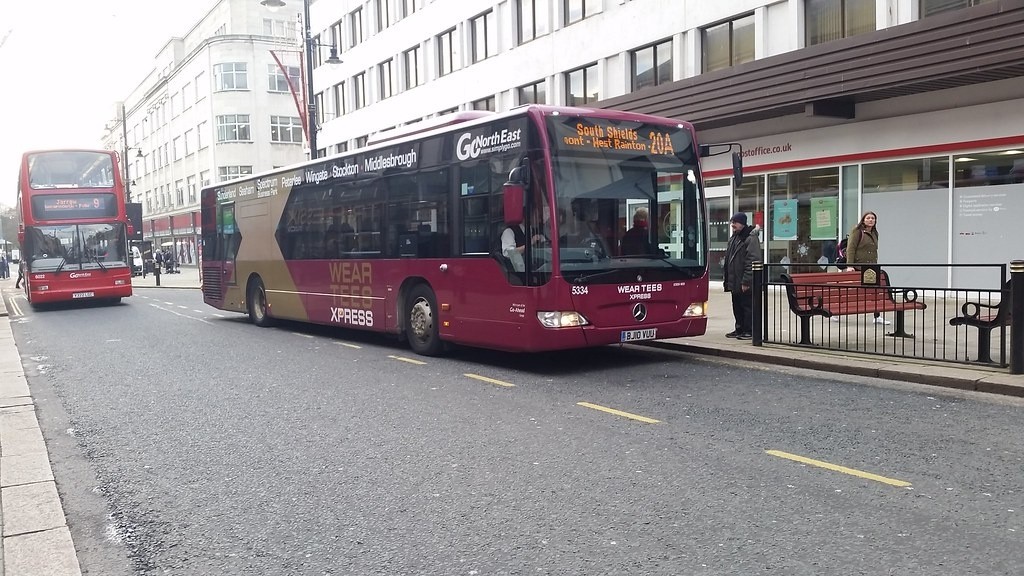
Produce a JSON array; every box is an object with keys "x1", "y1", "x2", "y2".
[
  {"x1": 873, "y1": 317, "x2": 890, "y2": 325},
  {"x1": 830, "y1": 316, "x2": 838, "y2": 321}
]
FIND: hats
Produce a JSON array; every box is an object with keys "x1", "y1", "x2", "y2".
[{"x1": 731, "y1": 212, "x2": 747, "y2": 225}]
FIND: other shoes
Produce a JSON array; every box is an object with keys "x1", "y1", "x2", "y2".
[
  {"x1": 736, "y1": 331, "x2": 752, "y2": 340},
  {"x1": 725, "y1": 330, "x2": 743, "y2": 338}
]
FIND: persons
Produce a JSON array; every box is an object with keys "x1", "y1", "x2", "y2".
[
  {"x1": 500, "y1": 207, "x2": 546, "y2": 286},
  {"x1": 350, "y1": 235, "x2": 370, "y2": 259},
  {"x1": 723, "y1": 212, "x2": 761, "y2": 339},
  {"x1": 2, "y1": 254, "x2": 7, "y2": 280},
  {"x1": 621, "y1": 207, "x2": 669, "y2": 258},
  {"x1": 832, "y1": 211, "x2": 891, "y2": 325},
  {"x1": 46, "y1": 239, "x2": 55, "y2": 253},
  {"x1": 154, "y1": 249, "x2": 162, "y2": 276},
  {"x1": 15, "y1": 259, "x2": 23, "y2": 288},
  {"x1": 163, "y1": 249, "x2": 170, "y2": 268}
]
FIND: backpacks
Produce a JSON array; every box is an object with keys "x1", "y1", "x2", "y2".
[{"x1": 832, "y1": 229, "x2": 878, "y2": 270}]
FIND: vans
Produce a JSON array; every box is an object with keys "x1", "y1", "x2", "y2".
[{"x1": 128, "y1": 246, "x2": 143, "y2": 276}]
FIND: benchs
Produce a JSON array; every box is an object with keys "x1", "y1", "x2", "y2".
[
  {"x1": 779, "y1": 269, "x2": 927, "y2": 346},
  {"x1": 948, "y1": 279, "x2": 1015, "y2": 367}
]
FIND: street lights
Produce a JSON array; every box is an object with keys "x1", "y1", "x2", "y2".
[
  {"x1": 125, "y1": 146, "x2": 143, "y2": 277},
  {"x1": 260, "y1": 0, "x2": 343, "y2": 161}
]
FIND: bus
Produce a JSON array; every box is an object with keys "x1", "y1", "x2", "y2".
[
  {"x1": 17, "y1": 149, "x2": 134, "y2": 306},
  {"x1": 200, "y1": 103, "x2": 742, "y2": 354}
]
